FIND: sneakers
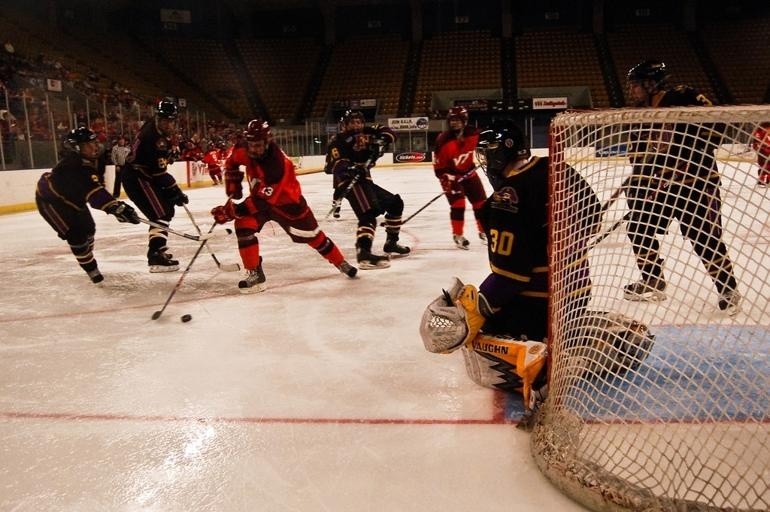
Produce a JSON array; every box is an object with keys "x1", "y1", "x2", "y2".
[
  {"x1": 80, "y1": 265, "x2": 104, "y2": 283},
  {"x1": 480, "y1": 233, "x2": 489, "y2": 245},
  {"x1": 147, "y1": 247, "x2": 172, "y2": 265},
  {"x1": 238, "y1": 256, "x2": 266, "y2": 289},
  {"x1": 453, "y1": 233, "x2": 469, "y2": 250},
  {"x1": 151, "y1": 253, "x2": 179, "y2": 272},
  {"x1": 338, "y1": 261, "x2": 357, "y2": 279}
]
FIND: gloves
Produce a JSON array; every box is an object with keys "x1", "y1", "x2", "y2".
[
  {"x1": 170, "y1": 186, "x2": 188, "y2": 206},
  {"x1": 210, "y1": 198, "x2": 243, "y2": 224},
  {"x1": 113, "y1": 202, "x2": 140, "y2": 224},
  {"x1": 346, "y1": 163, "x2": 370, "y2": 181},
  {"x1": 420, "y1": 278, "x2": 484, "y2": 355}
]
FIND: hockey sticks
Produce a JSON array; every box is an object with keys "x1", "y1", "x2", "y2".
[
  {"x1": 185, "y1": 204, "x2": 240, "y2": 273},
  {"x1": 289, "y1": 158, "x2": 373, "y2": 237},
  {"x1": 137, "y1": 216, "x2": 233, "y2": 241},
  {"x1": 381, "y1": 163, "x2": 486, "y2": 223},
  {"x1": 150, "y1": 219, "x2": 219, "y2": 322},
  {"x1": 268, "y1": 220, "x2": 285, "y2": 238}
]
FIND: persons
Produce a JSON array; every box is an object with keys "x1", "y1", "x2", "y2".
[
  {"x1": 121, "y1": 99, "x2": 189, "y2": 267},
  {"x1": 1, "y1": 38, "x2": 254, "y2": 201},
  {"x1": 325, "y1": 108, "x2": 411, "y2": 265},
  {"x1": 752, "y1": 121, "x2": 769, "y2": 188},
  {"x1": 618, "y1": 56, "x2": 741, "y2": 312},
  {"x1": 33, "y1": 125, "x2": 142, "y2": 283},
  {"x1": 322, "y1": 114, "x2": 349, "y2": 219},
  {"x1": 416, "y1": 119, "x2": 655, "y2": 426},
  {"x1": 210, "y1": 118, "x2": 358, "y2": 289},
  {"x1": 432, "y1": 104, "x2": 490, "y2": 246}
]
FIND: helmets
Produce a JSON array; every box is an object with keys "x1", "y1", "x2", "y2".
[
  {"x1": 245, "y1": 119, "x2": 270, "y2": 140},
  {"x1": 345, "y1": 109, "x2": 365, "y2": 122},
  {"x1": 67, "y1": 127, "x2": 99, "y2": 143},
  {"x1": 156, "y1": 101, "x2": 177, "y2": 119},
  {"x1": 476, "y1": 119, "x2": 531, "y2": 191},
  {"x1": 628, "y1": 59, "x2": 674, "y2": 108},
  {"x1": 447, "y1": 106, "x2": 468, "y2": 119}
]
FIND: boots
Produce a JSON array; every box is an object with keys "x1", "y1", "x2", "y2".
[
  {"x1": 383, "y1": 227, "x2": 410, "y2": 258},
  {"x1": 624, "y1": 253, "x2": 667, "y2": 300},
  {"x1": 355, "y1": 235, "x2": 391, "y2": 270},
  {"x1": 706, "y1": 253, "x2": 741, "y2": 310}
]
